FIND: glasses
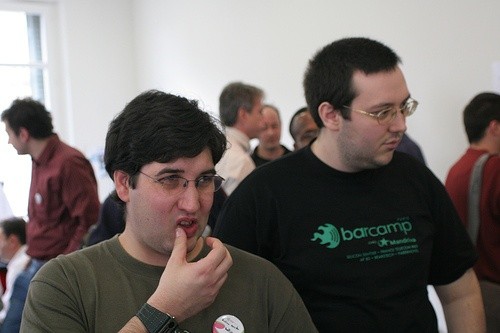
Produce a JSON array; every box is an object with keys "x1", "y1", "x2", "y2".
[
  {"x1": 342, "y1": 97, "x2": 419, "y2": 124},
  {"x1": 135, "y1": 169, "x2": 227, "y2": 195}
]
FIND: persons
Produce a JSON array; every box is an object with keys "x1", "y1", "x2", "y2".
[
  {"x1": 209, "y1": 38, "x2": 487, "y2": 333},
  {"x1": 0, "y1": 218, "x2": 30, "y2": 323},
  {"x1": 80, "y1": 83, "x2": 426, "y2": 252},
  {"x1": 0, "y1": 98, "x2": 101, "y2": 333},
  {"x1": 19, "y1": 89, "x2": 320, "y2": 333},
  {"x1": 444, "y1": 93, "x2": 500, "y2": 333}
]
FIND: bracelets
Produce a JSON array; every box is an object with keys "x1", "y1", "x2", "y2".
[{"x1": 136, "y1": 303, "x2": 184, "y2": 333}]
